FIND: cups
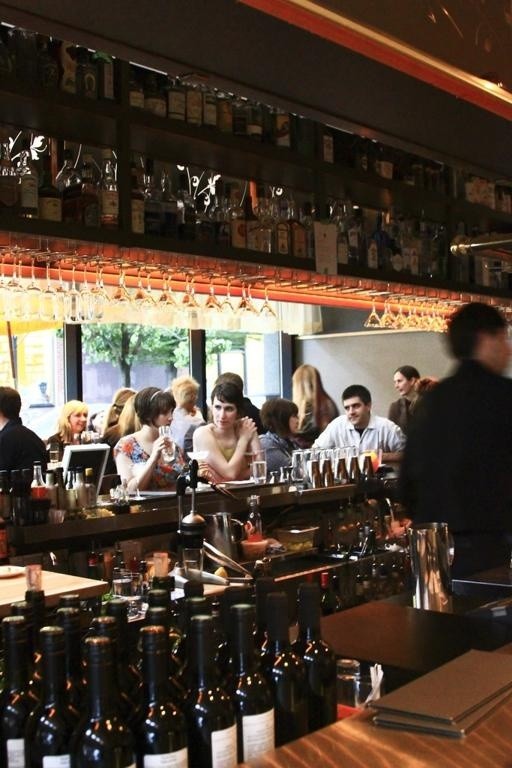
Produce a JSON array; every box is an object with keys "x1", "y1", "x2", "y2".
[
  {"x1": 407, "y1": 522, "x2": 455, "y2": 614},
  {"x1": 288, "y1": 446, "x2": 360, "y2": 491},
  {"x1": 252, "y1": 449, "x2": 268, "y2": 485},
  {"x1": 158, "y1": 426, "x2": 176, "y2": 462}
]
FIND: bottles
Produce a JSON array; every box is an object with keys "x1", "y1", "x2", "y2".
[
  {"x1": 0, "y1": 494, "x2": 398, "y2": 767},
  {"x1": 0, "y1": 27, "x2": 304, "y2": 271},
  {"x1": 0, "y1": 461, "x2": 97, "y2": 529},
  {"x1": 305, "y1": 118, "x2": 512, "y2": 296}
]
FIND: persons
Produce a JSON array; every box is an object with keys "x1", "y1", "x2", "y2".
[
  {"x1": 1, "y1": 366, "x2": 441, "y2": 492},
  {"x1": 397, "y1": 303, "x2": 511, "y2": 535}
]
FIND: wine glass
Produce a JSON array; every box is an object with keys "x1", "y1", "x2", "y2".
[
  {"x1": 0, "y1": 244, "x2": 281, "y2": 334},
  {"x1": 364, "y1": 297, "x2": 449, "y2": 333}
]
